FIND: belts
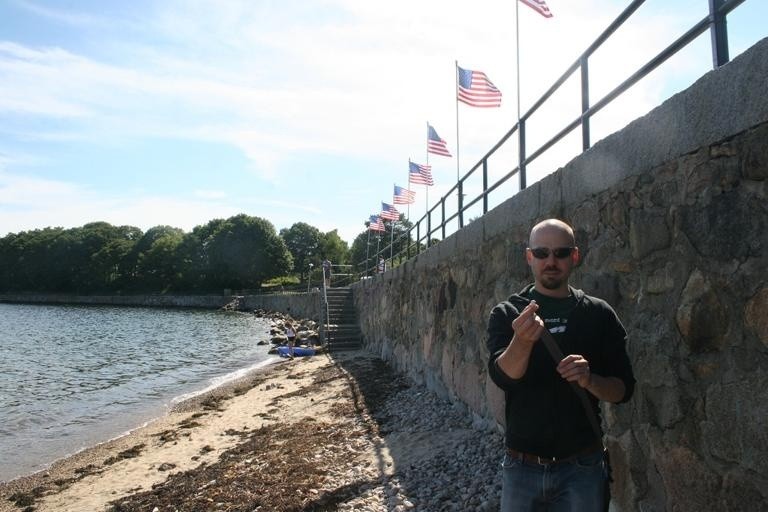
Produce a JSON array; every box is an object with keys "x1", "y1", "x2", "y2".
[{"x1": 508, "y1": 444, "x2": 600, "y2": 467}]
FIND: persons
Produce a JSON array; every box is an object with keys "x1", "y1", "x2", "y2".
[
  {"x1": 376, "y1": 254, "x2": 385, "y2": 275},
  {"x1": 247, "y1": 307, "x2": 320, "y2": 363},
  {"x1": 484, "y1": 217, "x2": 638, "y2": 511},
  {"x1": 321, "y1": 256, "x2": 332, "y2": 290}
]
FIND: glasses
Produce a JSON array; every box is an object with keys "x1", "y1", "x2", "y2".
[{"x1": 530, "y1": 247, "x2": 575, "y2": 259}]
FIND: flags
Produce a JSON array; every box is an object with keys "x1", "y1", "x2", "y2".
[
  {"x1": 380, "y1": 203, "x2": 400, "y2": 221},
  {"x1": 456, "y1": 65, "x2": 502, "y2": 108},
  {"x1": 369, "y1": 216, "x2": 386, "y2": 232},
  {"x1": 393, "y1": 185, "x2": 416, "y2": 204},
  {"x1": 517, "y1": 0, "x2": 557, "y2": 18},
  {"x1": 427, "y1": 124, "x2": 453, "y2": 158},
  {"x1": 409, "y1": 161, "x2": 434, "y2": 186}
]
